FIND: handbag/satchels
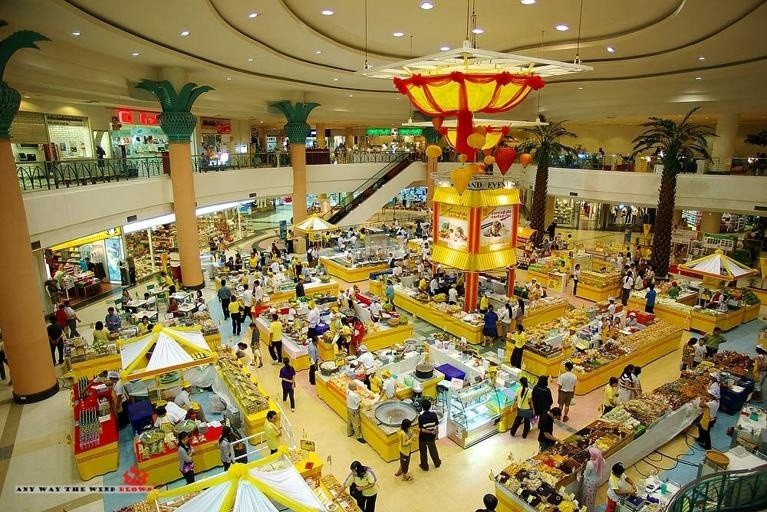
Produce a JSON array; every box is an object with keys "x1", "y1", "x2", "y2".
[
  {"x1": 571, "y1": 397, "x2": 576, "y2": 406},
  {"x1": 181, "y1": 464, "x2": 195, "y2": 473},
  {"x1": 518, "y1": 408, "x2": 533, "y2": 418},
  {"x1": 350, "y1": 483, "x2": 361, "y2": 499},
  {"x1": 531, "y1": 414, "x2": 538, "y2": 424}
]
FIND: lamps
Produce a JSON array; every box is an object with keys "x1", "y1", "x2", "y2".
[
  {"x1": 401, "y1": 30, "x2": 551, "y2": 159},
  {"x1": 356, "y1": 0, "x2": 594, "y2": 119}
]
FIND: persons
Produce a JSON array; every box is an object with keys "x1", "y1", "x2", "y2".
[
  {"x1": 85, "y1": 258, "x2": 96, "y2": 275},
  {"x1": 63, "y1": 301, "x2": 82, "y2": 337},
  {"x1": 93, "y1": 202, "x2": 728, "y2": 512},
  {"x1": 0, "y1": 334, "x2": 12, "y2": 385},
  {"x1": 751, "y1": 343, "x2": 767, "y2": 403},
  {"x1": 47, "y1": 315, "x2": 64, "y2": 366},
  {"x1": 56, "y1": 304, "x2": 70, "y2": 339},
  {"x1": 632, "y1": 210, "x2": 637, "y2": 224}
]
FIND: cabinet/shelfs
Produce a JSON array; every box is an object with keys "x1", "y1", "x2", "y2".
[{"x1": 44, "y1": 188, "x2": 767, "y2": 512}]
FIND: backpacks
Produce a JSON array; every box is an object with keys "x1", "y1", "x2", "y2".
[{"x1": 192, "y1": 303, "x2": 202, "y2": 312}]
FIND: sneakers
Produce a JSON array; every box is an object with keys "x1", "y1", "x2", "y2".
[
  {"x1": 435, "y1": 461, "x2": 441, "y2": 468},
  {"x1": 395, "y1": 471, "x2": 413, "y2": 482},
  {"x1": 251, "y1": 359, "x2": 282, "y2": 368},
  {"x1": 419, "y1": 464, "x2": 429, "y2": 471},
  {"x1": 694, "y1": 437, "x2": 710, "y2": 449},
  {"x1": 291, "y1": 407, "x2": 295, "y2": 412},
  {"x1": 562, "y1": 416, "x2": 568, "y2": 422},
  {"x1": 349, "y1": 430, "x2": 366, "y2": 444}
]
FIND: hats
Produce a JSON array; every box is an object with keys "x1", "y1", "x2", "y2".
[
  {"x1": 451, "y1": 284, "x2": 457, "y2": 288},
  {"x1": 629, "y1": 312, "x2": 635, "y2": 317},
  {"x1": 369, "y1": 369, "x2": 391, "y2": 379},
  {"x1": 672, "y1": 282, "x2": 677, "y2": 286},
  {"x1": 155, "y1": 400, "x2": 167, "y2": 409},
  {"x1": 183, "y1": 381, "x2": 191, "y2": 388},
  {"x1": 332, "y1": 288, "x2": 349, "y2": 314},
  {"x1": 710, "y1": 372, "x2": 720, "y2": 380},
  {"x1": 351, "y1": 346, "x2": 368, "y2": 365},
  {"x1": 708, "y1": 389, "x2": 720, "y2": 398},
  {"x1": 394, "y1": 261, "x2": 399, "y2": 266},
  {"x1": 109, "y1": 371, "x2": 119, "y2": 378},
  {"x1": 570, "y1": 325, "x2": 578, "y2": 331},
  {"x1": 308, "y1": 301, "x2": 315, "y2": 309},
  {"x1": 415, "y1": 260, "x2": 420, "y2": 264},
  {"x1": 609, "y1": 297, "x2": 615, "y2": 301},
  {"x1": 425, "y1": 275, "x2": 430, "y2": 279}
]
